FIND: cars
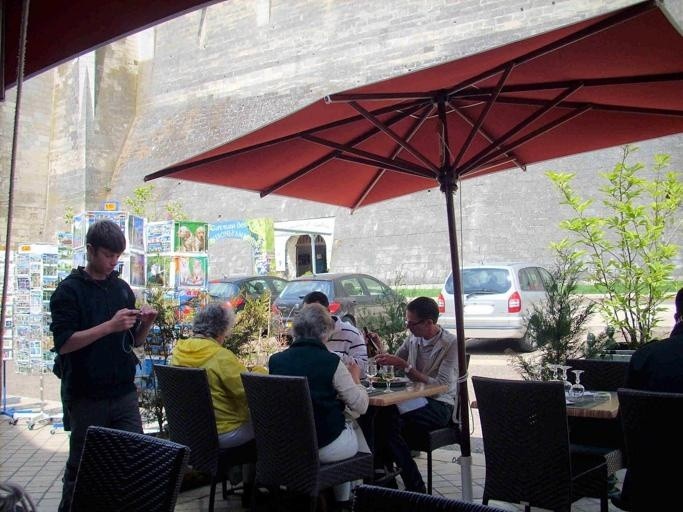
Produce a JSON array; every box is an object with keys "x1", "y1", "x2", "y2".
[
  {"x1": 437, "y1": 261, "x2": 563, "y2": 352},
  {"x1": 268, "y1": 271, "x2": 410, "y2": 346},
  {"x1": 207, "y1": 274, "x2": 287, "y2": 321}
]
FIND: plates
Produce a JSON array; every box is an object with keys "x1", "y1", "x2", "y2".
[{"x1": 365, "y1": 376, "x2": 410, "y2": 386}]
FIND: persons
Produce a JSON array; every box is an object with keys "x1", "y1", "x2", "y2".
[
  {"x1": 355, "y1": 298, "x2": 457, "y2": 492},
  {"x1": 303, "y1": 292, "x2": 367, "y2": 379},
  {"x1": 268, "y1": 304, "x2": 369, "y2": 511},
  {"x1": 170, "y1": 302, "x2": 267, "y2": 511},
  {"x1": 622, "y1": 286, "x2": 682, "y2": 395},
  {"x1": 51, "y1": 223, "x2": 156, "y2": 511}
]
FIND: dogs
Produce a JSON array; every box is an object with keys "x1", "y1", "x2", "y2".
[
  {"x1": 176, "y1": 226, "x2": 195, "y2": 253},
  {"x1": 192, "y1": 260, "x2": 204, "y2": 283},
  {"x1": 195, "y1": 227, "x2": 205, "y2": 252},
  {"x1": 179, "y1": 257, "x2": 192, "y2": 283}
]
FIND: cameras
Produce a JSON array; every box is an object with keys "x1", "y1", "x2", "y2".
[{"x1": 132, "y1": 309, "x2": 142, "y2": 320}]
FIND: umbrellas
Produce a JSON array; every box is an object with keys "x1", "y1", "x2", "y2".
[{"x1": 140, "y1": 0, "x2": 683, "y2": 502}]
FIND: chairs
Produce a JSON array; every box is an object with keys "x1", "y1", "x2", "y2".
[
  {"x1": 344, "y1": 283, "x2": 359, "y2": 295},
  {"x1": 254, "y1": 282, "x2": 265, "y2": 293}
]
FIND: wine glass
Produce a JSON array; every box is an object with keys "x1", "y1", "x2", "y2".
[
  {"x1": 382, "y1": 365, "x2": 394, "y2": 393},
  {"x1": 569, "y1": 368, "x2": 586, "y2": 399},
  {"x1": 548, "y1": 364, "x2": 561, "y2": 382},
  {"x1": 559, "y1": 366, "x2": 573, "y2": 396},
  {"x1": 365, "y1": 365, "x2": 377, "y2": 390}
]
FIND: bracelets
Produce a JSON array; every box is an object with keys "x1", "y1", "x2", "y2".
[{"x1": 403, "y1": 363, "x2": 418, "y2": 372}]
